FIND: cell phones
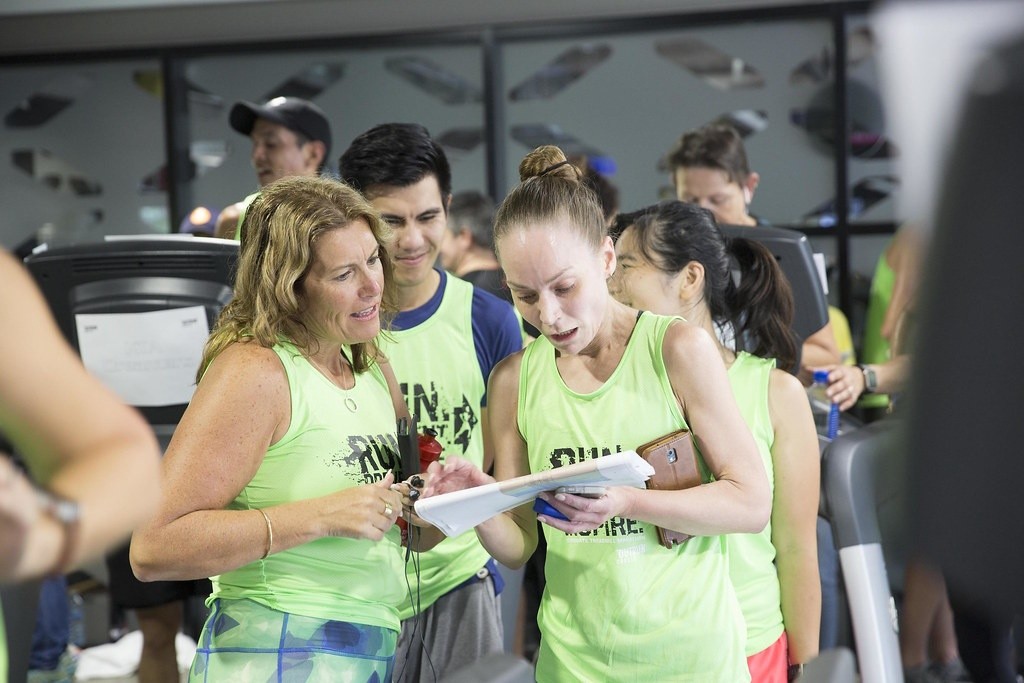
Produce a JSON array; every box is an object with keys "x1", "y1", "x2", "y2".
[
  {"x1": 396, "y1": 413, "x2": 419, "y2": 479},
  {"x1": 634, "y1": 428, "x2": 704, "y2": 549}
]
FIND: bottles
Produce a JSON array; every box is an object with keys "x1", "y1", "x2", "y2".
[{"x1": 809, "y1": 370, "x2": 842, "y2": 441}]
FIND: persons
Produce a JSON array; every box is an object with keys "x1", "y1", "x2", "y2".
[
  {"x1": 129, "y1": 173, "x2": 449, "y2": 683},
  {"x1": 672, "y1": 129, "x2": 843, "y2": 380},
  {"x1": 211, "y1": 99, "x2": 334, "y2": 242},
  {"x1": 0, "y1": 249, "x2": 163, "y2": 683},
  {"x1": 411, "y1": 144, "x2": 777, "y2": 680},
  {"x1": 335, "y1": 122, "x2": 526, "y2": 681},
  {"x1": 599, "y1": 202, "x2": 821, "y2": 683},
  {"x1": 894, "y1": 553, "x2": 973, "y2": 683},
  {"x1": 438, "y1": 188, "x2": 521, "y2": 306},
  {"x1": 804, "y1": 352, "x2": 912, "y2": 410}
]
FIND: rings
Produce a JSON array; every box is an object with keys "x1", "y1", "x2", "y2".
[{"x1": 381, "y1": 502, "x2": 395, "y2": 518}]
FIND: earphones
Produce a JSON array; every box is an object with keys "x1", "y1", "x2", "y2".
[
  {"x1": 406, "y1": 483, "x2": 420, "y2": 501},
  {"x1": 411, "y1": 476, "x2": 424, "y2": 488}
]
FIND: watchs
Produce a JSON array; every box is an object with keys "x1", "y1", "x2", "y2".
[
  {"x1": 39, "y1": 483, "x2": 83, "y2": 581},
  {"x1": 857, "y1": 363, "x2": 878, "y2": 394}
]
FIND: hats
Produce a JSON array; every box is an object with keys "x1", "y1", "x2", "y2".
[{"x1": 229, "y1": 95, "x2": 332, "y2": 153}]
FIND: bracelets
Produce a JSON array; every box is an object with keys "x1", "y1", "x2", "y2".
[
  {"x1": 254, "y1": 507, "x2": 274, "y2": 559},
  {"x1": 788, "y1": 664, "x2": 808, "y2": 680}
]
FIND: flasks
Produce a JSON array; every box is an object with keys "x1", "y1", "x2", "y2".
[{"x1": 395, "y1": 425, "x2": 440, "y2": 527}]
